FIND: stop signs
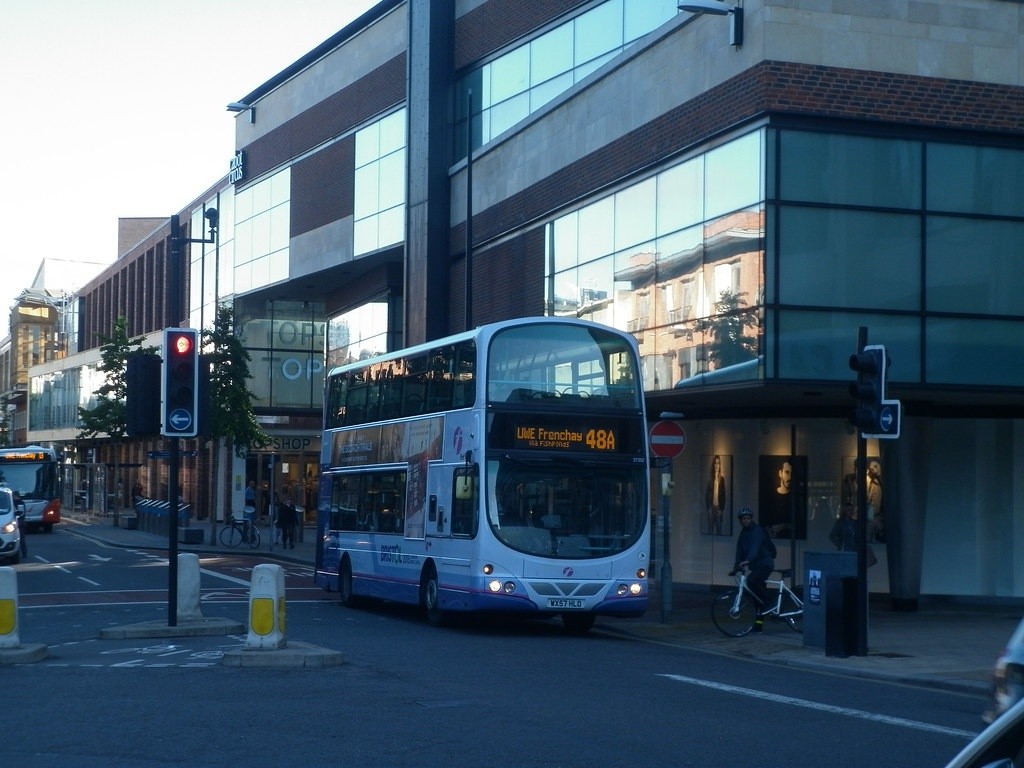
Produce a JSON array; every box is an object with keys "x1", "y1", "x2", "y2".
[{"x1": 649, "y1": 420, "x2": 684, "y2": 459}]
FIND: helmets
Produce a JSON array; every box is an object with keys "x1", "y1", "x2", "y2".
[{"x1": 738, "y1": 508, "x2": 753, "y2": 517}]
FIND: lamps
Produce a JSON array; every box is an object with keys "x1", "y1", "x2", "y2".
[
  {"x1": 676, "y1": 0, "x2": 744, "y2": 46},
  {"x1": 227, "y1": 102, "x2": 256, "y2": 124}
]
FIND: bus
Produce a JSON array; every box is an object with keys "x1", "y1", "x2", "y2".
[
  {"x1": 0, "y1": 445, "x2": 62, "y2": 534},
  {"x1": 316, "y1": 314, "x2": 676, "y2": 634},
  {"x1": 338, "y1": 489, "x2": 399, "y2": 531}
]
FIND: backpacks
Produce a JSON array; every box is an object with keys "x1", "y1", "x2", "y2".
[{"x1": 765, "y1": 529, "x2": 777, "y2": 558}]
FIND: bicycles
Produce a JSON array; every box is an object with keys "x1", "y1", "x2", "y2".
[
  {"x1": 219, "y1": 515, "x2": 261, "y2": 551},
  {"x1": 711, "y1": 559, "x2": 804, "y2": 639}
]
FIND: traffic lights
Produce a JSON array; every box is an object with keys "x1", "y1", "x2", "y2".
[
  {"x1": 160, "y1": 326, "x2": 199, "y2": 437},
  {"x1": 847, "y1": 345, "x2": 886, "y2": 433}
]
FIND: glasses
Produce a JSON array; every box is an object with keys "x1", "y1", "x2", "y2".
[{"x1": 743, "y1": 517, "x2": 751, "y2": 520}]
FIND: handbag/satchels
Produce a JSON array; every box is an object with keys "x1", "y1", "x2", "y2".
[{"x1": 275, "y1": 519, "x2": 280, "y2": 529}]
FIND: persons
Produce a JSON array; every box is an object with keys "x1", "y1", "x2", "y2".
[
  {"x1": 386, "y1": 425, "x2": 398, "y2": 462},
  {"x1": 735, "y1": 508, "x2": 776, "y2": 635},
  {"x1": 279, "y1": 497, "x2": 298, "y2": 549},
  {"x1": 759, "y1": 460, "x2": 794, "y2": 537},
  {"x1": 867, "y1": 458, "x2": 882, "y2": 507},
  {"x1": 245, "y1": 481, "x2": 255, "y2": 506},
  {"x1": 706, "y1": 456, "x2": 726, "y2": 535},
  {"x1": 261, "y1": 482, "x2": 271, "y2": 516},
  {"x1": 273, "y1": 495, "x2": 282, "y2": 543},
  {"x1": 829, "y1": 498, "x2": 881, "y2": 552}
]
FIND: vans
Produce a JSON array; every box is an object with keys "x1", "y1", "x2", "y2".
[{"x1": 0, "y1": 487, "x2": 25, "y2": 561}]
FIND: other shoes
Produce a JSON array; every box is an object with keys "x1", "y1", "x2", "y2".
[
  {"x1": 742, "y1": 628, "x2": 763, "y2": 635},
  {"x1": 290, "y1": 544, "x2": 295, "y2": 549},
  {"x1": 760, "y1": 600, "x2": 777, "y2": 615},
  {"x1": 283, "y1": 545, "x2": 287, "y2": 550}
]
